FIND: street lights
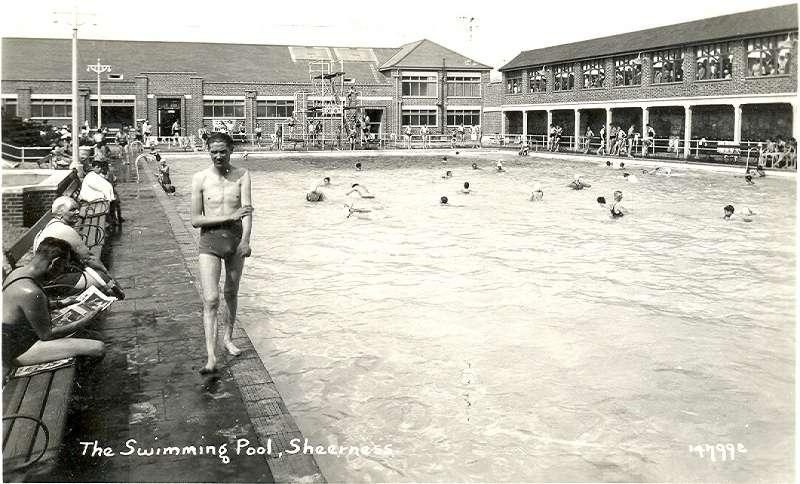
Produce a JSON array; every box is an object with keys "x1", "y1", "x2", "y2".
[
  {"x1": 52, "y1": 6, "x2": 97, "y2": 174},
  {"x1": 85, "y1": 59, "x2": 111, "y2": 132}
]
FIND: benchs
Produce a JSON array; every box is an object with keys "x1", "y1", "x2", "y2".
[{"x1": 2, "y1": 167, "x2": 123, "y2": 483}]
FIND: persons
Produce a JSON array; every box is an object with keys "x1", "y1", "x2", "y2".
[
  {"x1": 31, "y1": 196, "x2": 112, "y2": 298},
  {"x1": 190, "y1": 132, "x2": 256, "y2": 376},
  {"x1": 3, "y1": 236, "x2": 107, "y2": 369},
  {"x1": 348, "y1": 86, "x2": 359, "y2": 107},
  {"x1": 515, "y1": 121, "x2": 797, "y2": 222},
  {"x1": 2, "y1": 106, "x2": 187, "y2": 224},
  {"x1": 304, "y1": 161, "x2": 506, "y2": 219},
  {"x1": 196, "y1": 115, "x2": 487, "y2": 160},
  {"x1": 519, "y1": 44, "x2": 789, "y2": 93}
]
